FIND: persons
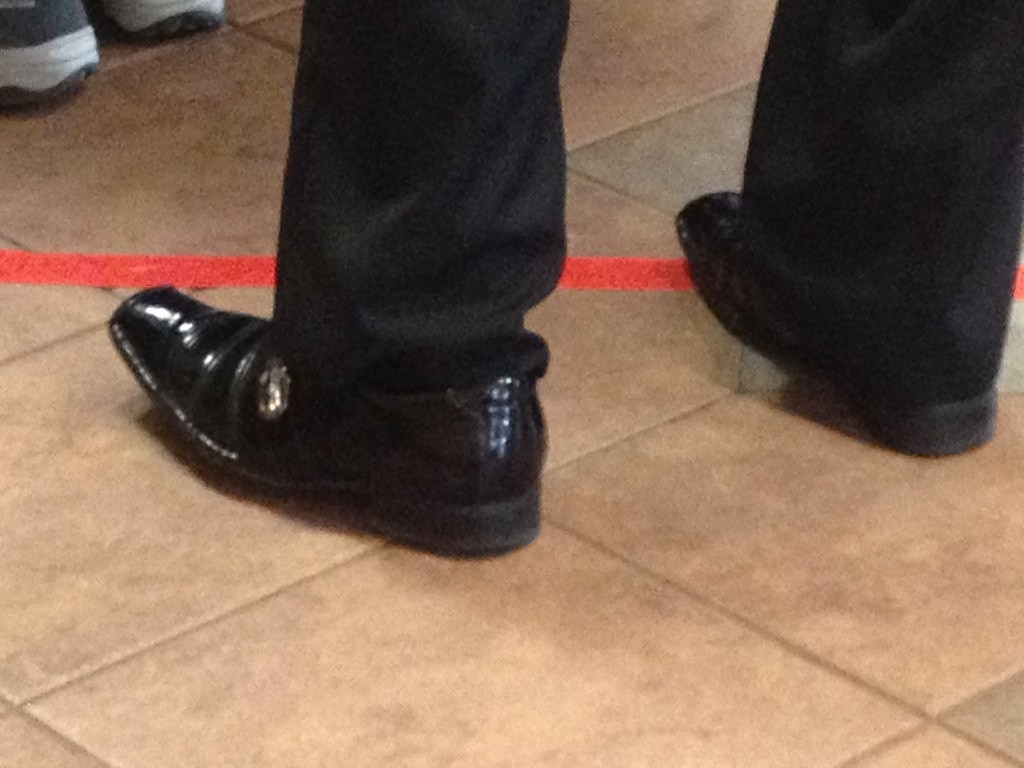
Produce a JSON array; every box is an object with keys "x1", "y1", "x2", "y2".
[{"x1": 105, "y1": 0, "x2": 1024, "y2": 558}]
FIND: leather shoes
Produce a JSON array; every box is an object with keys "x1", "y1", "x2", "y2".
[
  {"x1": 677, "y1": 193, "x2": 996, "y2": 452},
  {"x1": 106, "y1": 287, "x2": 545, "y2": 559}
]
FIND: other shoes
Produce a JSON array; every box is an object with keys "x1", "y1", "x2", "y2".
[
  {"x1": 0, "y1": 0, "x2": 98, "y2": 113},
  {"x1": 90, "y1": 0, "x2": 223, "y2": 41}
]
